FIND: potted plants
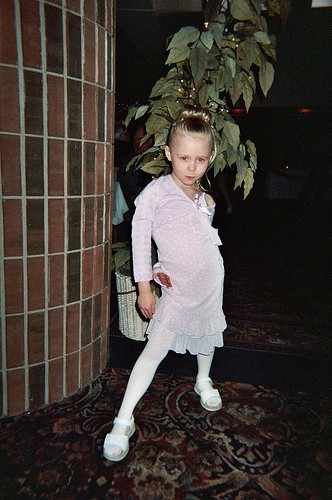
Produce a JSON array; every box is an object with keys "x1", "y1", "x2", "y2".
[{"x1": 112, "y1": 0, "x2": 283, "y2": 344}]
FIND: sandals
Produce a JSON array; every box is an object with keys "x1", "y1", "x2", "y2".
[
  {"x1": 193, "y1": 376, "x2": 223, "y2": 411},
  {"x1": 103, "y1": 414, "x2": 136, "y2": 462}
]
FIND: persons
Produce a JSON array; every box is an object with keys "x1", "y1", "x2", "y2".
[{"x1": 103, "y1": 118, "x2": 225, "y2": 462}]
